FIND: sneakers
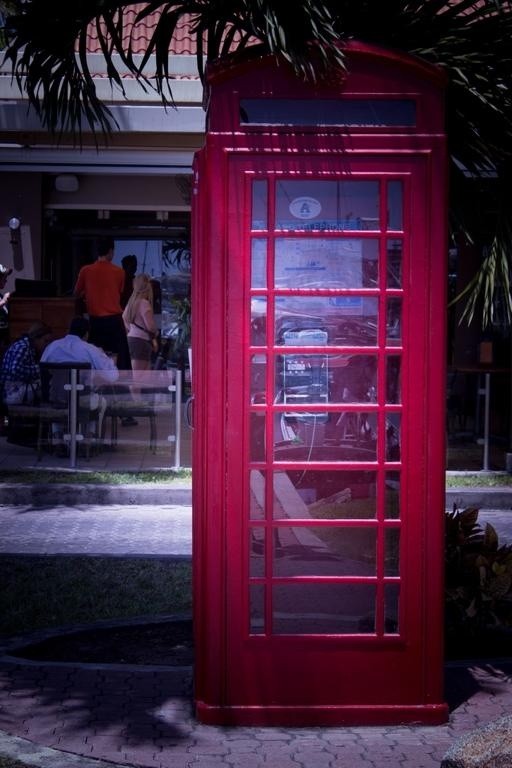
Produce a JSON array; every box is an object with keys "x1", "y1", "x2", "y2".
[{"x1": 122, "y1": 417, "x2": 138, "y2": 426}]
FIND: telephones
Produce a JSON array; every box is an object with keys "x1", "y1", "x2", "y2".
[{"x1": 277, "y1": 331, "x2": 328, "y2": 418}]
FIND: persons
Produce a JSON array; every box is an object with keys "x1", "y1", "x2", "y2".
[
  {"x1": 120, "y1": 252, "x2": 142, "y2": 311},
  {"x1": 72, "y1": 237, "x2": 141, "y2": 427},
  {"x1": 40, "y1": 317, "x2": 123, "y2": 450},
  {"x1": 1, "y1": 322, "x2": 57, "y2": 447},
  {"x1": 0, "y1": 262, "x2": 13, "y2": 438},
  {"x1": 120, "y1": 271, "x2": 162, "y2": 407}
]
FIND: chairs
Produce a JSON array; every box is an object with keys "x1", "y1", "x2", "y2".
[{"x1": 5, "y1": 360, "x2": 182, "y2": 473}]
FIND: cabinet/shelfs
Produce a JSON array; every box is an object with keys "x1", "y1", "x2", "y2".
[{"x1": 9, "y1": 297, "x2": 80, "y2": 359}]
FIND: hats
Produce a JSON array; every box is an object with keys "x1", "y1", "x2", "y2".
[{"x1": 0, "y1": 264, "x2": 13, "y2": 275}]
